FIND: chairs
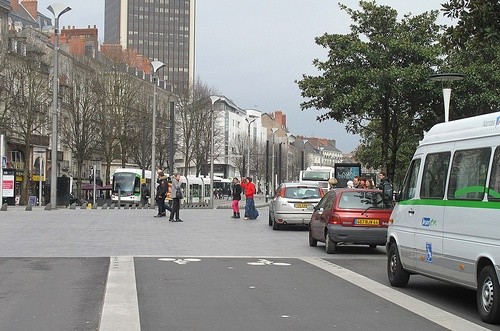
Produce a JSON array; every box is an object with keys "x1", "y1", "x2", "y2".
[{"x1": 305, "y1": 190, "x2": 313, "y2": 197}]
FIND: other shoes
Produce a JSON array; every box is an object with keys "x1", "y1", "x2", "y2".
[
  {"x1": 254, "y1": 215, "x2": 259, "y2": 219},
  {"x1": 168, "y1": 218, "x2": 176, "y2": 222},
  {"x1": 243, "y1": 216, "x2": 250, "y2": 220},
  {"x1": 154, "y1": 213, "x2": 162, "y2": 217},
  {"x1": 175, "y1": 219, "x2": 183, "y2": 222}
]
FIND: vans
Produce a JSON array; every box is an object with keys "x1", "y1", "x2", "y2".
[{"x1": 386, "y1": 112, "x2": 500, "y2": 324}]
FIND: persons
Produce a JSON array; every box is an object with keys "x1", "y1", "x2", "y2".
[
  {"x1": 213, "y1": 186, "x2": 231, "y2": 200},
  {"x1": 240, "y1": 177, "x2": 259, "y2": 220},
  {"x1": 347, "y1": 176, "x2": 376, "y2": 189},
  {"x1": 376, "y1": 172, "x2": 392, "y2": 190},
  {"x1": 154, "y1": 170, "x2": 172, "y2": 217},
  {"x1": 141, "y1": 183, "x2": 150, "y2": 210},
  {"x1": 168, "y1": 172, "x2": 183, "y2": 222},
  {"x1": 329, "y1": 178, "x2": 338, "y2": 189},
  {"x1": 231, "y1": 177, "x2": 242, "y2": 218}
]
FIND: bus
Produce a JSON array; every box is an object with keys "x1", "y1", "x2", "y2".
[
  {"x1": 299, "y1": 165, "x2": 335, "y2": 195},
  {"x1": 111, "y1": 168, "x2": 233, "y2": 206}
]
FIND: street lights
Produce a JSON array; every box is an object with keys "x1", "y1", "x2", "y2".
[
  {"x1": 244, "y1": 117, "x2": 255, "y2": 177},
  {"x1": 286, "y1": 133, "x2": 292, "y2": 181},
  {"x1": 150, "y1": 60, "x2": 166, "y2": 209},
  {"x1": 319, "y1": 146, "x2": 325, "y2": 167},
  {"x1": 270, "y1": 127, "x2": 279, "y2": 197},
  {"x1": 209, "y1": 95, "x2": 222, "y2": 209},
  {"x1": 302, "y1": 139, "x2": 308, "y2": 172},
  {"x1": 429, "y1": 73, "x2": 466, "y2": 123},
  {"x1": 46, "y1": 2, "x2": 72, "y2": 210}
]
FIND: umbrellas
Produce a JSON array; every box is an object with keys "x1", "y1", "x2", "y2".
[{"x1": 81, "y1": 184, "x2": 113, "y2": 200}]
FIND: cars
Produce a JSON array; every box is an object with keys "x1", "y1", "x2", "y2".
[
  {"x1": 265, "y1": 182, "x2": 325, "y2": 231},
  {"x1": 307, "y1": 189, "x2": 395, "y2": 253}
]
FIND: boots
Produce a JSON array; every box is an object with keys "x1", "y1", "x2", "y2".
[{"x1": 230, "y1": 211, "x2": 241, "y2": 218}]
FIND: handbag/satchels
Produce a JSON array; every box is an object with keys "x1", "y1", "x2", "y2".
[
  {"x1": 176, "y1": 186, "x2": 183, "y2": 199},
  {"x1": 248, "y1": 199, "x2": 259, "y2": 219}
]
